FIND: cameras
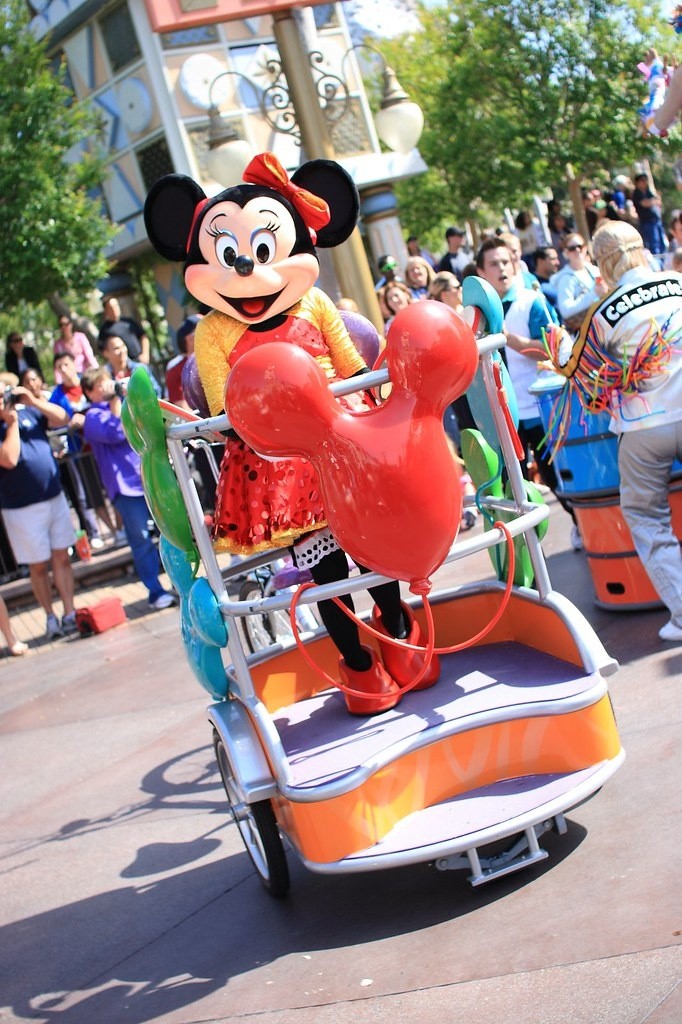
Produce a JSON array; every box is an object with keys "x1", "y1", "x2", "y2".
[
  {"x1": 2, "y1": 386, "x2": 21, "y2": 407},
  {"x1": 115, "y1": 376, "x2": 130, "y2": 393}
]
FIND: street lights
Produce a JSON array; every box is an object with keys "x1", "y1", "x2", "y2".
[{"x1": 206, "y1": 8, "x2": 425, "y2": 336}]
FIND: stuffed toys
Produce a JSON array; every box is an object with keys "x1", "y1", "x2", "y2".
[{"x1": 143, "y1": 152, "x2": 439, "y2": 713}]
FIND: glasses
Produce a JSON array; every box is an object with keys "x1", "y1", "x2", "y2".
[
  {"x1": 12, "y1": 338, "x2": 22, "y2": 342},
  {"x1": 454, "y1": 284, "x2": 463, "y2": 290},
  {"x1": 565, "y1": 244, "x2": 585, "y2": 253},
  {"x1": 381, "y1": 260, "x2": 400, "y2": 273}
]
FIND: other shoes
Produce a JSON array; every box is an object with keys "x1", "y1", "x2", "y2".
[
  {"x1": 659, "y1": 621, "x2": 682, "y2": 641},
  {"x1": 571, "y1": 525, "x2": 583, "y2": 551}
]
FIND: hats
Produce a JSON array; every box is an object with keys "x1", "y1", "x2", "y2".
[{"x1": 445, "y1": 227, "x2": 466, "y2": 238}]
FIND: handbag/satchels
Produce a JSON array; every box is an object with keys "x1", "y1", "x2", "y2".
[{"x1": 76, "y1": 598, "x2": 127, "y2": 638}]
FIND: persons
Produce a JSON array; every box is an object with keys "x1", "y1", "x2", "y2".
[
  {"x1": 0, "y1": 295, "x2": 225, "y2": 656},
  {"x1": 375, "y1": 174, "x2": 682, "y2": 454},
  {"x1": 548, "y1": 220, "x2": 682, "y2": 641},
  {"x1": 637, "y1": 4, "x2": 682, "y2": 141},
  {"x1": 475, "y1": 239, "x2": 582, "y2": 550}
]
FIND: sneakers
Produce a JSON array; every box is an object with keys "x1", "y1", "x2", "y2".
[
  {"x1": 148, "y1": 591, "x2": 177, "y2": 609},
  {"x1": 61, "y1": 610, "x2": 75, "y2": 636},
  {"x1": 46, "y1": 615, "x2": 64, "y2": 640}
]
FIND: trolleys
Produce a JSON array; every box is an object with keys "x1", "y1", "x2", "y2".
[{"x1": 164, "y1": 334, "x2": 626, "y2": 898}]
